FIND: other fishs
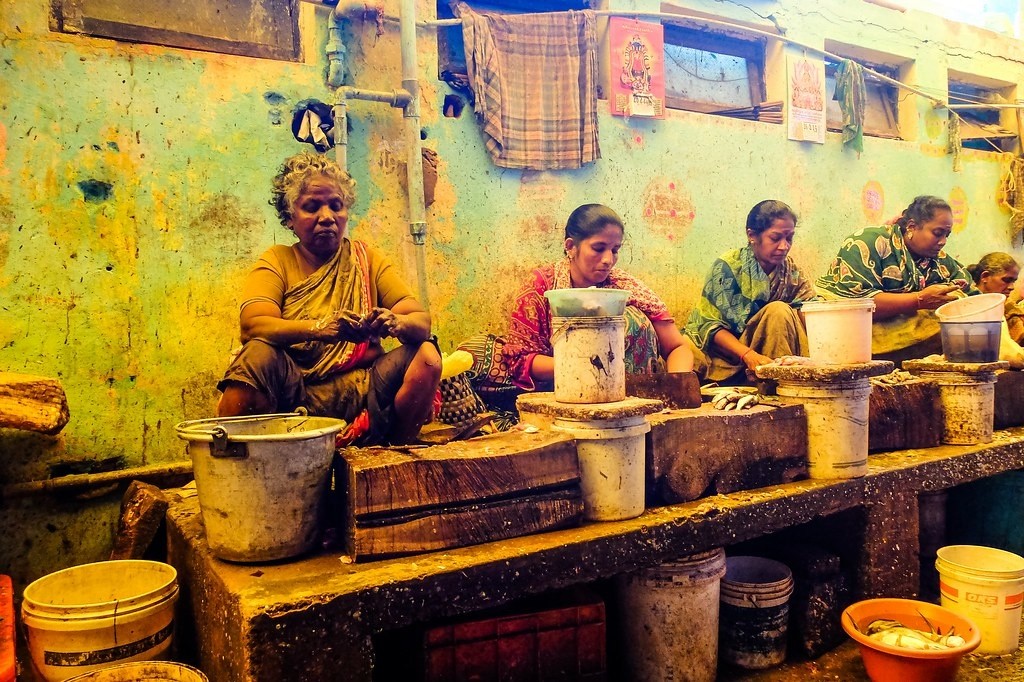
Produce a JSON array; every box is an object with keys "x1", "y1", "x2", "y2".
[
  {"x1": 711, "y1": 390, "x2": 759, "y2": 411},
  {"x1": 846, "y1": 610, "x2": 966, "y2": 652}
]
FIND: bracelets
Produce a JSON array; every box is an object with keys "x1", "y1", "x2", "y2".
[
  {"x1": 740, "y1": 349, "x2": 752, "y2": 360},
  {"x1": 916, "y1": 293, "x2": 922, "y2": 309}
]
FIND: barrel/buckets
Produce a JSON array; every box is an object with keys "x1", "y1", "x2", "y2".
[
  {"x1": 916, "y1": 370, "x2": 994, "y2": 446},
  {"x1": 617, "y1": 546, "x2": 726, "y2": 682},
  {"x1": 21, "y1": 558, "x2": 178, "y2": 682},
  {"x1": 776, "y1": 377, "x2": 870, "y2": 478},
  {"x1": 934, "y1": 544, "x2": 1024, "y2": 654},
  {"x1": 802, "y1": 299, "x2": 876, "y2": 366},
  {"x1": 59, "y1": 660, "x2": 210, "y2": 682},
  {"x1": 553, "y1": 416, "x2": 651, "y2": 520},
  {"x1": 550, "y1": 317, "x2": 626, "y2": 404},
  {"x1": 720, "y1": 556, "x2": 792, "y2": 669},
  {"x1": 175, "y1": 406, "x2": 345, "y2": 563}
]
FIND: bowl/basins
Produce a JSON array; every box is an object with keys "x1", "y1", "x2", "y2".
[
  {"x1": 935, "y1": 293, "x2": 1006, "y2": 322},
  {"x1": 938, "y1": 320, "x2": 1003, "y2": 362},
  {"x1": 544, "y1": 288, "x2": 631, "y2": 317},
  {"x1": 840, "y1": 597, "x2": 982, "y2": 681}
]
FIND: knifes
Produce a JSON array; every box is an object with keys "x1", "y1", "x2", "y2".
[{"x1": 370, "y1": 443, "x2": 428, "y2": 450}]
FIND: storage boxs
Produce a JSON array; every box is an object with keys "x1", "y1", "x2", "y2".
[{"x1": 422, "y1": 599, "x2": 607, "y2": 682}]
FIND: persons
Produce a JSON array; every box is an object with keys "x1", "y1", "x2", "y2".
[
  {"x1": 966, "y1": 252, "x2": 1024, "y2": 345},
  {"x1": 813, "y1": 194, "x2": 982, "y2": 371},
  {"x1": 500, "y1": 203, "x2": 694, "y2": 394},
  {"x1": 215, "y1": 154, "x2": 444, "y2": 445},
  {"x1": 680, "y1": 199, "x2": 822, "y2": 394}
]
